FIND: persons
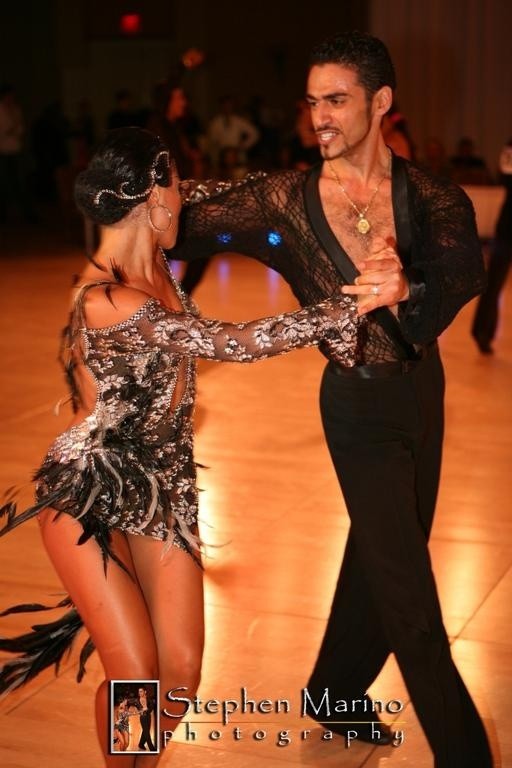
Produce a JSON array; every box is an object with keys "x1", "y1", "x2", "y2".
[
  {"x1": 471, "y1": 142, "x2": 512, "y2": 354},
  {"x1": 114, "y1": 687, "x2": 155, "y2": 751},
  {"x1": 36, "y1": 126, "x2": 403, "y2": 768},
  {"x1": 0, "y1": 45, "x2": 487, "y2": 295},
  {"x1": 169, "y1": 33, "x2": 492, "y2": 767}
]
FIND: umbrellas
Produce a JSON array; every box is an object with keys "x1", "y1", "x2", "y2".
[{"x1": 304, "y1": 694, "x2": 391, "y2": 745}]
